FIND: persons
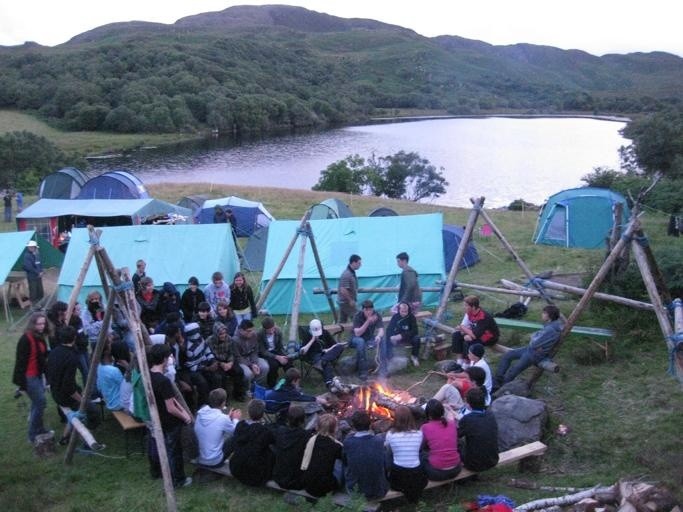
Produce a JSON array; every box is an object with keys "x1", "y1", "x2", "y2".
[
  {"x1": 20, "y1": 241, "x2": 45, "y2": 310},
  {"x1": 212, "y1": 205, "x2": 226, "y2": 224},
  {"x1": 13, "y1": 192, "x2": 23, "y2": 213},
  {"x1": 223, "y1": 208, "x2": 237, "y2": 248},
  {"x1": 44, "y1": 251, "x2": 501, "y2": 506},
  {"x1": 2, "y1": 190, "x2": 14, "y2": 223},
  {"x1": 11, "y1": 312, "x2": 56, "y2": 448},
  {"x1": 490, "y1": 304, "x2": 562, "y2": 396}
]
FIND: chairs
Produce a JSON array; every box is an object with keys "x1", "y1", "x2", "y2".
[{"x1": 298, "y1": 325, "x2": 344, "y2": 387}]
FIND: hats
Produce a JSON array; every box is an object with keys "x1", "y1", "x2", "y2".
[
  {"x1": 309, "y1": 319, "x2": 322, "y2": 337},
  {"x1": 25, "y1": 241, "x2": 39, "y2": 249},
  {"x1": 469, "y1": 344, "x2": 484, "y2": 357}
]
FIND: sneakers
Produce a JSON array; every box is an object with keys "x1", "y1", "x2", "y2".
[
  {"x1": 410, "y1": 354, "x2": 419, "y2": 367},
  {"x1": 28, "y1": 430, "x2": 54, "y2": 444},
  {"x1": 359, "y1": 372, "x2": 367, "y2": 380},
  {"x1": 232, "y1": 390, "x2": 245, "y2": 402},
  {"x1": 312, "y1": 364, "x2": 323, "y2": 371},
  {"x1": 182, "y1": 477, "x2": 192, "y2": 486},
  {"x1": 34, "y1": 436, "x2": 44, "y2": 449},
  {"x1": 245, "y1": 389, "x2": 252, "y2": 398},
  {"x1": 461, "y1": 363, "x2": 469, "y2": 370}
]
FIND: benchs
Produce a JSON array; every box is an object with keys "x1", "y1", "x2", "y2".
[
  {"x1": 110, "y1": 409, "x2": 147, "y2": 461},
  {"x1": 493, "y1": 317, "x2": 612, "y2": 360}
]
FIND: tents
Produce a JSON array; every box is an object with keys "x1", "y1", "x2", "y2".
[
  {"x1": 527, "y1": 186, "x2": 631, "y2": 252},
  {"x1": 15, "y1": 199, "x2": 191, "y2": 252},
  {"x1": 191, "y1": 196, "x2": 278, "y2": 238},
  {"x1": 73, "y1": 169, "x2": 151, "y2": 200},
  {"x1": 35, "y1": 165, "x2": 87, "y2": 200},
  {"x1": 302, "y1": 198, "x2": 353, "y2": 221},
  {"x1": 366, "y1": 206, "x2": 400, "y2": 217},
  {"x1": 442, "y1": 224, "x2": 482, "y2": 276},
  {"x1": 177, "y1": 194, "x2": 214, "y2": 215},
  {"x1": 241, "y1": 227, "x2": 267, "y2": 273},
  {"x1": 54, "y1": 211, "x2": 450, "y2": 314}
]
